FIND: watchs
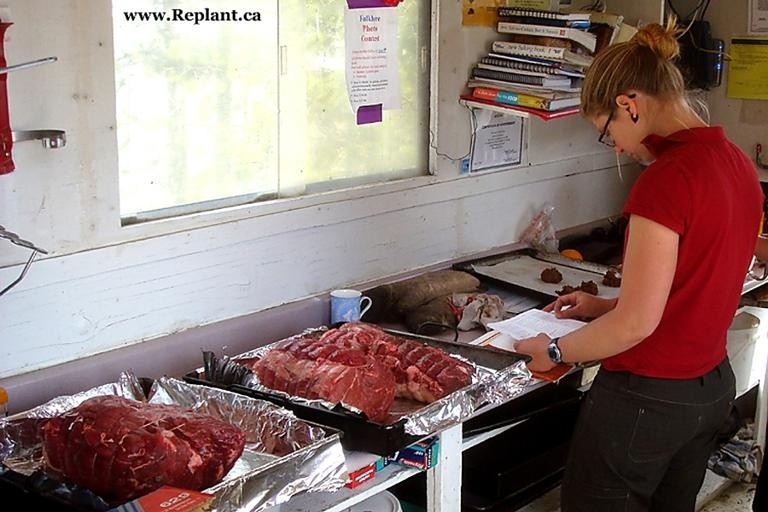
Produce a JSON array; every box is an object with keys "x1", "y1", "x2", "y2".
[{"x1": 547, "y1": 338, "x2": 565, "y2": 365}]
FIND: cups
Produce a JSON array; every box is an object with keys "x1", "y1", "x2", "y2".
[{"x1": 329, "y1": 288, "x2": 373, "y2": 324}]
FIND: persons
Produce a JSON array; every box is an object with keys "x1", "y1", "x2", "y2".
[
  {"x1": 514, "y1": 23, "x2": 765, "y2": 512},
  {"x1": 755, "y1": 234, "x2": 768, "y2": 260}
]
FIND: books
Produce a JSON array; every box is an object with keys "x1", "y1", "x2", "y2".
[{"x1": 460, "y1": 7, "x2": 624, "y2": 121}]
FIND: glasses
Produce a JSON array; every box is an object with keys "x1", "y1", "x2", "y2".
[{"x1": 597, "y1": 94, "x2": 636, "y2": 147}]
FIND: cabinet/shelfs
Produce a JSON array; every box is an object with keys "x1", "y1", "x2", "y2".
[{"x1": 329, "y1": 277, "x2": 767, "y2": 511}]
